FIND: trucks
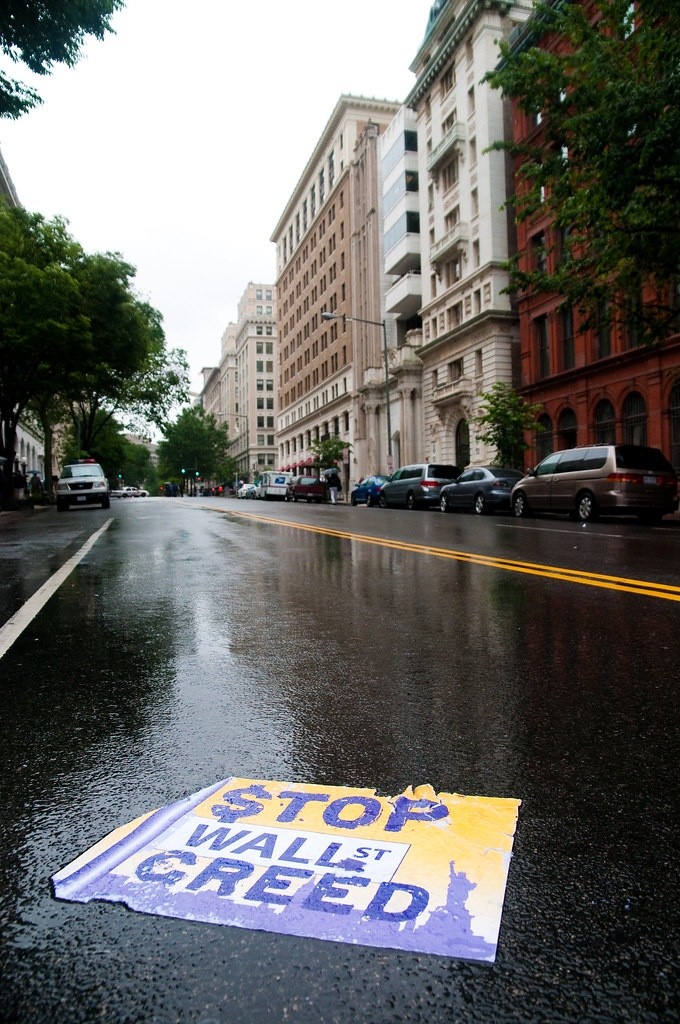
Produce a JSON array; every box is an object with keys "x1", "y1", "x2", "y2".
[{"x1": 253, "y1": 469, "x2": 292, "y2": 502}]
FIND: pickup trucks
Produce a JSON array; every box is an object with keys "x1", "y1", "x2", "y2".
[{"x1": 110, "y1": 487, "x2": 149, "y2": 498}]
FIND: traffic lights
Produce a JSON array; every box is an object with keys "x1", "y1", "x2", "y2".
[
  {"x1": 160, "y1": 487, "x2": 163, "y2": 490},
  {"x1": 119, "y1": 475, "x2": 121, "y2": 479},
  {"x1": 196, "y1": 472, "x2": 200, "y2": 481},
  {"x1": 181, "y1": 468, "x2": 185, "y2": 473}
]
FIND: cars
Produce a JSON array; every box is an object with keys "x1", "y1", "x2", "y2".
[
  {"x1": 438, "y1": 465, "x2": 525, "y2": 515},
  {"x1": 54, "y1": 459, "x2": 111, "y2": 511},
  {"x1": 285, "y1": 475, "x2": 327, "y2": 503},
  {"x1": 350, "y1": 474, "x2": 392, "y2": 507},
  {"x1": 237, "y1": 483, "x2": 257, "y2": 499}
]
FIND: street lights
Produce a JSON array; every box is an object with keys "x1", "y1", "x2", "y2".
[
  {"x1": 321, "y1": 312, "x2": 392, "y2": 474},
  {"x1": 217, "y1": 412, "x2": 250, "y2": 483},
  {"x1": 180, "y1": 454, "x2": 198, "y2": 496}
]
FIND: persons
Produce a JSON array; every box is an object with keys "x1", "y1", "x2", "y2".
[
  {"x1": 29, "y1": 473, "x2": 44, "y2": 499},
  {"x1": 14, "y1": 469, "x2": 30, "y2": 500},
  {"x1": 171, "y1": 483, "x2": 178, "y2": 497},
  {"x1": 179, "y1": 482, "x2": 185, "y2": 497},
  {"x1": 165, "y1": 484, "x2": 171, "y2": 497},
  {"x1": 324, "y1": 472, "x2": 343, "y2": 505}
]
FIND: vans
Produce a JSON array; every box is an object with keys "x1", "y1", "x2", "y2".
[
  {"x1": 509, "y1": 441, "x2": 680, "y2": 523},
  {"x1": 379, "y1": 462, "x2": 462, "y2": 510}
]
